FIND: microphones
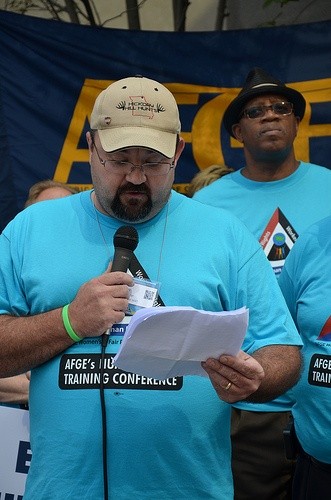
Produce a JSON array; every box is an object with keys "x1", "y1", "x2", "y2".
[{"x1": 101, "y1": 225, "x2": 138, "y2": 346}]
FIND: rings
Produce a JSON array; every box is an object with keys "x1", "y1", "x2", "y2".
[{"x1": 224, "y1": 382, "x2": 232, "y2": 393}]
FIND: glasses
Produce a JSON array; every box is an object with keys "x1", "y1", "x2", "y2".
[
  {"x1": 92, "y1": 134, "x2": 179, "y2": 177},
  {"x1": 238, "y1": 102, "x2": 297, "y2": 124}
]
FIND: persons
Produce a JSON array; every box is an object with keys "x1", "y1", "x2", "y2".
[
  {"x1": 0, "y1": 181, "x2": 74, "y2": 500},
  {"x1": 0, "y1": 76, "x2": 303, "y2": 500},
  {"x1": 279, "y1": 216, "x2": 331, "y2": 500},
  {"x1": 189, "y1": 166, "x2": 231, "y2": 197},
  {"x1": 193, "y1": 71, "x2": 331, "y2": 500}
]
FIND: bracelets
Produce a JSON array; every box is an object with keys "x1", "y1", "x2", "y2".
[{"x1": 62, "y1": 303, "x2": 83, "y2": 343}]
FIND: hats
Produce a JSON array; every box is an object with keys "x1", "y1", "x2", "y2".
[
  {"x1": 222, "y1": 70, "x2": 305, "y2": 139},
  {"x1": 89, "y1": 74, "x2": 181, "y2": 159}
]
